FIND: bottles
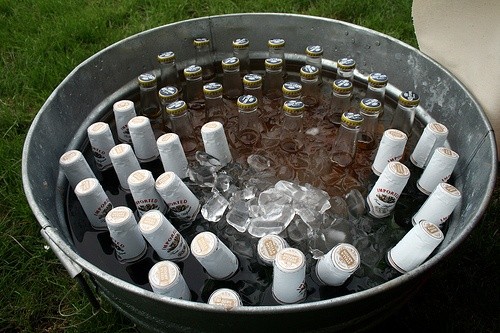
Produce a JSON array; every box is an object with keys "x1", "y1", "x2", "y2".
[{"x1": 59, "y1": 37, "x2": 462, "y2": 308}]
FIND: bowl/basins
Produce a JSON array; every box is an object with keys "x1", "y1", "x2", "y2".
[{"x1": 22, "y1": 12, "x2": 497, "y2": 332}]
